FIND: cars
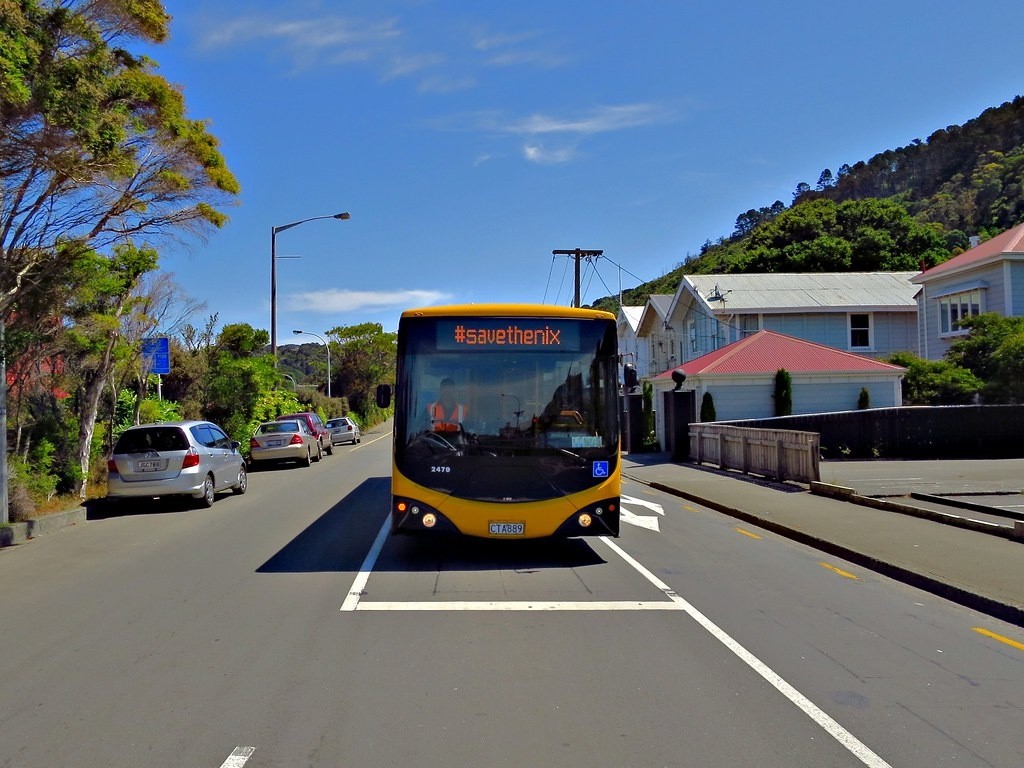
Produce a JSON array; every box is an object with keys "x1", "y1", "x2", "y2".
[
  {"x1": 249, "y1": 419, "x2": 318, "y2": 467},
  {"x1": 105, "y1": 421, "x2": 247, "y2": 508},
  {"x1": 325, "y1": 416, "x2": 361, "y2": 444}
]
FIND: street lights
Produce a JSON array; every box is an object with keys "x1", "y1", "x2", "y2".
[
  {"x1": 271, "y1": 212, "x2": 350, "y2": 417},
  {"x1": 292, "y1": 331, "x2": 331, "y2": 397}
]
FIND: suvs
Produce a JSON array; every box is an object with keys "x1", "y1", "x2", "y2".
[{"x1": 274, "y1": 412, "x2": 333, "y2": 458}]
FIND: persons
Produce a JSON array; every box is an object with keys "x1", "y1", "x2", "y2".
[
  {"x1": 542, "y1": 380, "x2": 580, "y2": 422},
  {"x1": 415, "y1": 377, "x2": 470, "y2": 432}
]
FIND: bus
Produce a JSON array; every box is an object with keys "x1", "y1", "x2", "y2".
[{"x1": 376, "y1": 302, "x2": 639, "y2": 541}]
